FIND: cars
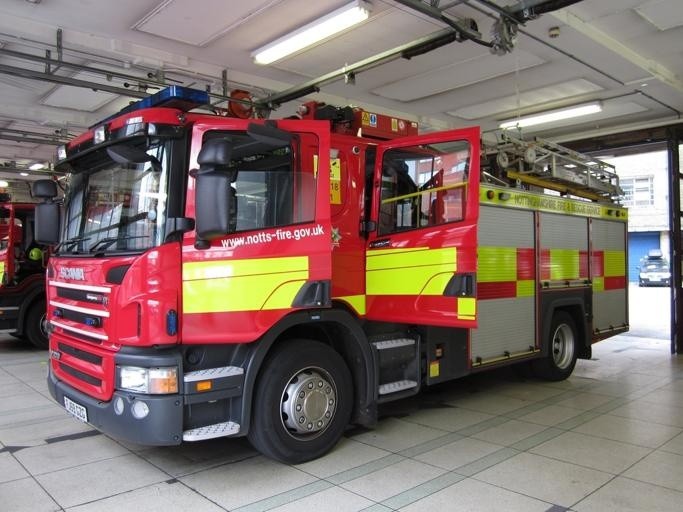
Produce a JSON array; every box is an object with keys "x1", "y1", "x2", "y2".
[{"x1": 635, "y1": 249, "x2": 672, "y2": 287}]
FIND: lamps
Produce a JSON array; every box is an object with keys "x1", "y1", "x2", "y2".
[
  {"x1": 496, "y1": 99, "x2": 602, "y2": 130},
  {"x1": 251, "y1": 0, "x2": 372, "y2": 67}
]
